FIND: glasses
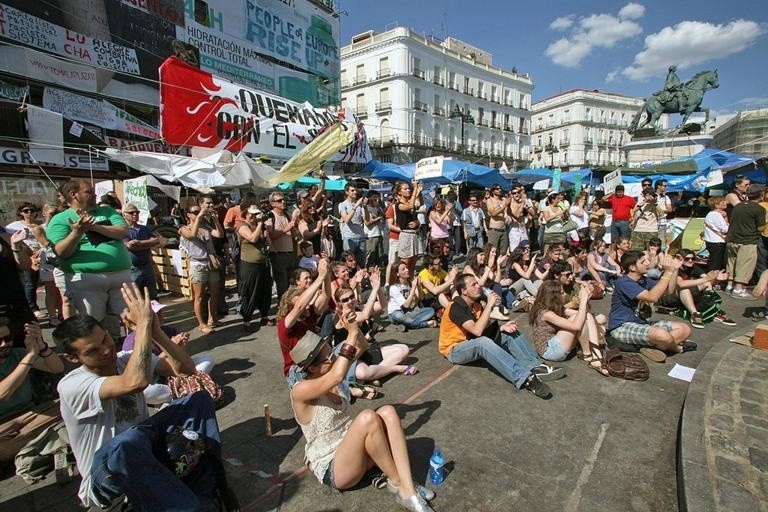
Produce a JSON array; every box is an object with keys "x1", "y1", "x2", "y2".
[
  {"x1": 123, "y1": 209, "x2": 142, "y2": 216},
  {"x1": 18, "y1": 207, "x2": 36, "y2": 214},
  {"x1": 642, "y1": 182, "x2": 651, "y2": 189},
  {"x1": 0, "y1": 333, "x2": 14, "y2": 348},
  {"x1": 188, "y1": 209, "x2": 200, "y2": 217},
  {"x1": 493, "y1": 187, "x2": 503, "y2": 191},
  {"x1": 559, "y1": 272, "x2": 574, "y2": 277},
  {"x1": 317, "y1": 350, "x2": 337, "y2": 368},
  {"x1": 272, "y1": 197, "x2": 288, "y2": 204},
  {"x1": 682, "y1": 255, "x2": 696, "y2": 264},
  {"x1": 335, "y1": 293, "x2": 355, "y2": 302},
  {"x1": 511, "y1": 191, "x2": 521, "y2": 195}
]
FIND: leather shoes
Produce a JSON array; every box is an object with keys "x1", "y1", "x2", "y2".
[
  {"x1": 241, "y1": 323, "x2": 250, "y2": 333},
  {"x1": 260, "y1": 318, "x2": 274, "y2": 328}
]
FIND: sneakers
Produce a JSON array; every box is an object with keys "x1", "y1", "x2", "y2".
[
  {"x1": 725, "y1": 284, "x2": 757, "y2": 300},
  {"x1": 712, "y1": 311, "x2": 737, "y2": 327},
  {"x1": 384, "y1": 477, "x2": 436, "y2": 512},
  {"x1": 678, "y1": 338, "x2": 698, "y2": 353},
  {"x1": 641, "y1": 346, "x2": 666, "y2": 365},
  {"x1": 523, "y1": 376, "x2": 553, "y2": 401},
  {"x1": 391, "y1": 324, "x2": 407, "y2": 331},
  {"x1": 511, "y1": 300, "x2": 529, "y2": 313},
  {"x1": 490, "y1": 306, "x2": 512, "y2": 321},
  {"x1": 535, "y1": 365, "x2": 567, "y2": 380},
  {"x1": 687, "y1": 311, "x2": 703, "y2": 331},
  {"x1": 426, "y1": 318, "x2": 438, "y2": 326}
]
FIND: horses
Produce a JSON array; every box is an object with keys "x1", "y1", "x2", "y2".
[{"x1": 627, "y1": 67, "x2": 720, "y2": 137}]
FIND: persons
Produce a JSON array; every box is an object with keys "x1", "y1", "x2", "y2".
[
  {"x1": 289, "y1": 310, "x2": 437, "y2": 512},
  {"x1": 663, "y1": 64, "x2": 690, "y2": 113},
  {"x1": 730, "y1": 175, "x2": 764, "y2": 301}
]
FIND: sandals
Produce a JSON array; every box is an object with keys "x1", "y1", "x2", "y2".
[
  {"x1": 196, "y1": 323, "x2": 216, "y2": 334},
  {"x1": 47, "y1": 313, "x2": 61, "y2": 327},
  {"x1": 578, "y1": 341, "x2": 609, "y2": 380},
  {"x1": 398, "y1": 363, "x2": 419, "y2": 375},
  {"x1": 210, "y1": 321, "x2": 226, "y2": 327}
]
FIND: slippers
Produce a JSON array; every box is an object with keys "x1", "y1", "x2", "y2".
[{"x1": 351, "y1": 381, "x2": 379, "y2": 404}]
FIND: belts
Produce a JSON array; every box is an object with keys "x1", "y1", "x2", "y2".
[
  {"x1": 472, "y1": 226, "x2": 482, "y2": 233},
  {"x1": 269, "y1": 250, "x2": 295, "y2": 257},
  {"x1": 402, "y1": 229, "x2": 421, "y2": 236},
  {"x1": 488, "y1": 226, "x2": 507, "y2": 234}
]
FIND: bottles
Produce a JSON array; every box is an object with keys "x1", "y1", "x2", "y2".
[
  {"x1": 428, "y1": 448, "x2": 445, "y2": 487},
  {"x1": 480, "y1": 286, "x2": 501, "y2": 306}
]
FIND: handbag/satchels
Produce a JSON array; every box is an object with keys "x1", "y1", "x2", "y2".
[
  {"x1": 587, "y1": 281, "x2": 603, "y2": 300},
  {"x1": 602, "y1": 351, "x2": 650, "y2": 380},
  {"x1": 674, "y1": 288, "x2": 722, "y2": 323},
  {"x1": 206, "y1": 247, "x2": 223, "y2": 271},
  {"x1": 168, "y1": 372, "x2": 225, "y2": 406},
  {"x1": 561, "y1": 215, "x2": 579, "y2": 233}
]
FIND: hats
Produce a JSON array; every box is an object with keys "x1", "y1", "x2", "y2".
[
  {"x1": 148, "y1": 298, "x2": 167, "y2": 313},
  {"x1": 288, "y1": 328, "x2": 332, "y2": 373},
  {"x1": 248, "y1": 205, "x2": 261, "y2": 217}
]
